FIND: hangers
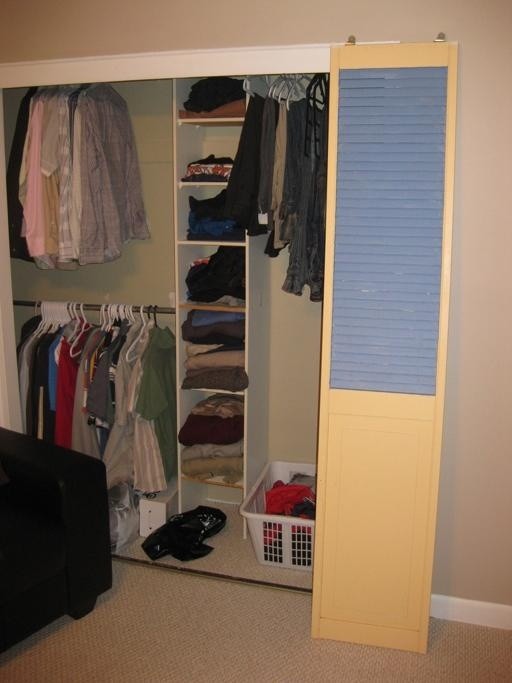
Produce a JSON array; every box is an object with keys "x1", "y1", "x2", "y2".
[
  {"x1": 32, "y1": 299, "x2": 160, "y2": 365},
  {"x1": 243, "y1": 74, "x2": 330, "y2": 104}
]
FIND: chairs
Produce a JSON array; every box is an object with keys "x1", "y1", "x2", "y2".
[
  {"x1": 0, "y1": 427, "x2": 113, "y2": 650},
  {"x1": 0, "y1": 38, "x2": 459, "y2": 654}
]
